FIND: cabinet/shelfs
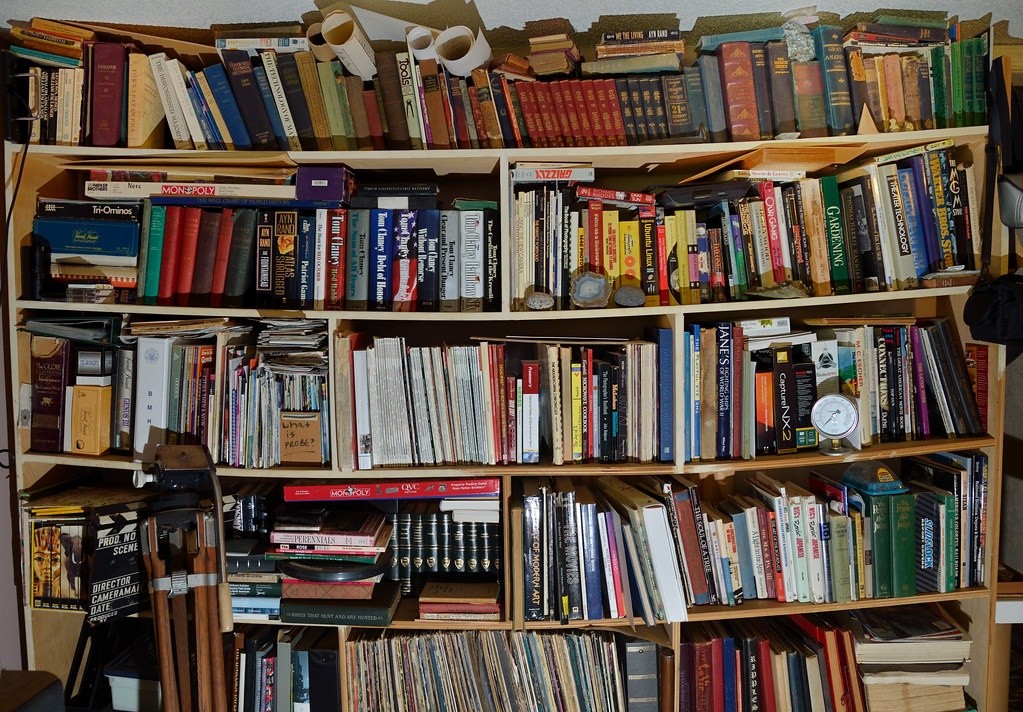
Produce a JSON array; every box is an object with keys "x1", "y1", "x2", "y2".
[{"x1": 0, "y1": 126, "x2": 1023, "y2": 712}]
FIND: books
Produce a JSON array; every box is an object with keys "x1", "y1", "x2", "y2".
[
  {"x1": 1, "y1": 7, "x2": 998, "y2": 148},
  {"x1": 19, "y1": 307, "x2": 985, "y2": 466},
  {"x1": 235, "y1": 631, "x2": 984, "y2": 711},
  {"x1": 23, "y1": 478, "x2": 987, "y2": 631},
  {"x1": 32, "y1": 144, "x2": 988, "y2": 313}
]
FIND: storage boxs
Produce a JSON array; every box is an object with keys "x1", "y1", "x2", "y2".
[
  {"x1": 77, "y1": 352, "x2": 102, "y2": 374},
  {"x1": 295, "y1": 163, "x2": 355, "y2": 203},
  {"x1": 31, "y1": 210, "x2": 140, "y2": 258},
  {"x1": 70, "y1": 387, "x2": 110, "y2": 456},
  {"x1": 281, "y1": 410, "x2": 323, "y2": 463}
]
